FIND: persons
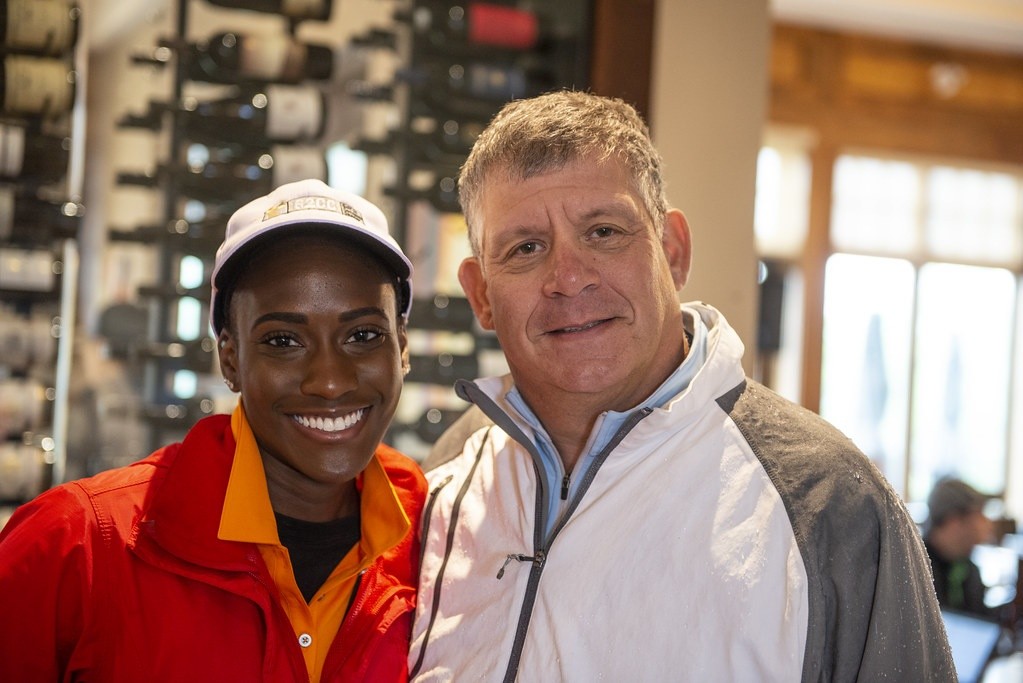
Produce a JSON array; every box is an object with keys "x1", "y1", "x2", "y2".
[
  {"x1": 406, "y1": 90, "x2": 958, "y2": 683},
  {"x1": 921, "y1": 479, "x2": 1003, "y2": 664},
  {"x1": 1, "y1": 178, "x2": 429, "y2": 683}
]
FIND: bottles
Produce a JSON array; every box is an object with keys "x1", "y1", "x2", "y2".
[{"x1": 0, "y1": 0, "x2": 588, "y2": 540}]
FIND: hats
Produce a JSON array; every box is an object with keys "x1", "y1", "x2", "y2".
[
  {"x1": 928, "y1": 476, "x2": 1004, "y2": 518},
  {"x1": 209, "y1": 179, "x2": 413, "y2": 339}
]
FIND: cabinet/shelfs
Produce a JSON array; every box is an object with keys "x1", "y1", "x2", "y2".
[{"x1": 0, "y1": 0, "x2": 598, "y2": 491}]
